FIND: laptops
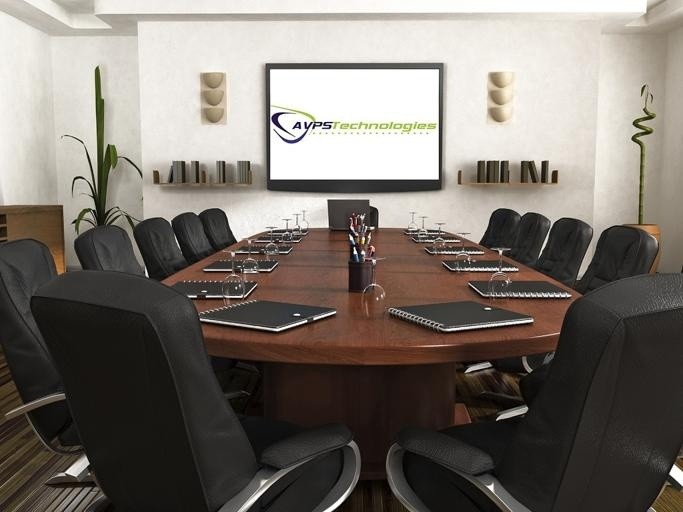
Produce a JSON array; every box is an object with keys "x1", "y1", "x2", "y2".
[{"x1": 328, "y1": 200, "x2": 375, "y2": 230}]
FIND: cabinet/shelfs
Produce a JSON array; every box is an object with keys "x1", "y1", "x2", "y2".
[{"x1": 0, "y1": 204, "x2": 65, "y2": 274}]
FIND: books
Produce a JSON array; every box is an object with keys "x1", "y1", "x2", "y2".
[
  {"x1": 254, "y1": 235, "x2": 303, "y2": 243},
  {"x1": 201, "y1": 260, "x2": 279, "y2": 273},
  {"x1": 166, "y1": 159, "x2": 250, "y2": 182},
  {"x1": 412, "y1": 237, "x2": 461, "y2": 243},
  {"x1": 441, "y1": 260, "x2": 521, "y2": 274},
  {"x1": 467, "y1": 279, "x2": 572, "y2": 301},
  {"x1": 477, "y1": 159, "x2": 549, "y2": 184},
  {"x1": 403, "y1": 230, "x2": 445, "y2": 235},
  {"x1": 230, "y1": 244, "x2": 293, "y2": 256},
  {"x1": 384, "y1": 300, "x2": 535, "y2": 333},
  {"x1": 423, "y1": 244, "x2": 485, "y2": 256},
  {"x1": 169, "y1": 279, "x2": 258, "y2": 299},
  {"x1": 195, "y1": 300, "x2": 338, "y2": 334},
  {"x1": 267, "y1": 230, "x2": 312, "y2": 235}
]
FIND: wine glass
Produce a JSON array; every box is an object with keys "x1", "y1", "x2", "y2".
[
  {"x1": 263, "y1": 224, "x2": 279, "y2": 256},
  {"x1": 281, "y1": 217, "x2": 292, "y2": 243},
  {"x1": 239, "y1": 237, "x2": 258, "y2": 274},
  {"x1": 298, "y1": 209, "x2": 312, "y2": 229},
  {"x1": 489, "y1": 247, "x2": 512, "y2": 304},
  {"x1": 221, "y1": 247, "x2": 245, "y2": 305},
  {"x1": 416, "y1": 216, "x2": 429, "y2": 239},
  {"x1": 291, "y1": 212, "x2": 302, "y2": 235},
  {"x1": 360, "y1": 255, "x2": 387, "y2": 317},
  {"x1": 431, "y1": 222, "x2": 447, "y2": 255},
  {"x1": 453, "y1": 231, "x2": 473, "y2": 274},
  {"x1": 406, "y1": 210, "x2": 418, "y2": 231}
]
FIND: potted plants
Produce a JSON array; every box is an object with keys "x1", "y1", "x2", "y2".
[{"x1": 622, "y1": 81, "x2": 666, "y2": 275}]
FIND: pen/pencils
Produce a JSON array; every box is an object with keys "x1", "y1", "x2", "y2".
[{"x1": 348, "y1": 212, "x2": 375, "y2": 263}]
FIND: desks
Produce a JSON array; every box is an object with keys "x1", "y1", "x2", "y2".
[{"x1": 142, "y1": 223, "x2": 593, "y2": 512}]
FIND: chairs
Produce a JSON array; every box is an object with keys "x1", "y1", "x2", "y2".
[
  {"x1": 28, "y1": 268, "x2": 363, "y2": 512},
  {"x1": 369, "y1": 206, "x2": 380, "y2": 231},
  {"x1": 0, "y1": 234, "x2": 101, "y2": 489},
  {"x1": 382, "y1": 269, "x2": 683, "y2": 512},
  {"x1": 473, "y1": 207, "x2": 662, "y2": 296},
  {"x1": 72, "y1": 207, "x2": 238, "y2": 284}
]
